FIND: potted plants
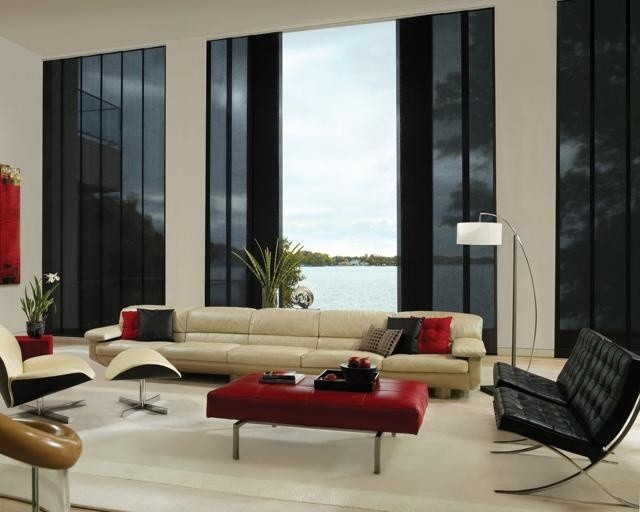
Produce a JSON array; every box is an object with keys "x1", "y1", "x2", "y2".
[{"x1": 231, "y1": 237, "x2": 306, "y2": 308}]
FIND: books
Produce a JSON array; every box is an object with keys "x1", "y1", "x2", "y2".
[
  {"x1": 263, "y1": 369, "x2": 296, "y2": 380},
  {"x1": 259, "y1": 373, "x2": 305, "y2": 385}
]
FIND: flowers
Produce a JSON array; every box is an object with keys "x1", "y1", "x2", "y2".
[{"x1": 20, "y1": 272, "x2": 61, "y2": 322}]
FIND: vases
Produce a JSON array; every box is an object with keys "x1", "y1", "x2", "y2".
[{"x1": 27, "y1": 319, "x2": 47, "y2": 338}]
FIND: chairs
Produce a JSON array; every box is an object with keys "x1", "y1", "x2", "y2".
[{"x1": 1, "y1": 325, "x2": 96, "y2": 423}]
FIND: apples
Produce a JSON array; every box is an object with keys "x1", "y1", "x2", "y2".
[
  {"x1": 358, "y1": 358, "x2": 371, "y2": 368},
  {"x1": 325, "y1": 374, "x2": 336, "y2": 381},
  {"x1": 349, "y1": 357, "x2": 361, "y2": 368}
]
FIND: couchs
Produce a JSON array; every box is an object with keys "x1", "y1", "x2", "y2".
[{"x1": 84, "y1": 304, "x2": 486, "y2": 399}]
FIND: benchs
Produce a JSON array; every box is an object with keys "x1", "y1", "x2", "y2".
[{"x1": 491, "y1": 328, "x2": 640, "y2": 509}]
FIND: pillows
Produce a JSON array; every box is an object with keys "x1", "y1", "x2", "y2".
[
  {"x1": 359, "y1": 315, "x2": 454, "y2": 358},
  {"x1": 120, "y1": 308, "x2": 176, "y2": 343}
]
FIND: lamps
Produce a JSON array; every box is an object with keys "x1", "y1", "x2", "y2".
[{"x1": 456, "y1": 212, "x2": 537, "y2": 396}]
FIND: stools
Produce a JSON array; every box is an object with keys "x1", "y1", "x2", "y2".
[
  {"x1": 104, "y1": 347, "x2": 182, "y2": 419},
  {"x1": 15, "y1": 334, "x2": 54, "y2": 362}
]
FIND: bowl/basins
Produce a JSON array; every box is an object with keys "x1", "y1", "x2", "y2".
[{"x1": 340, "y1": 362, "x2": 377, "y2": 382}]
